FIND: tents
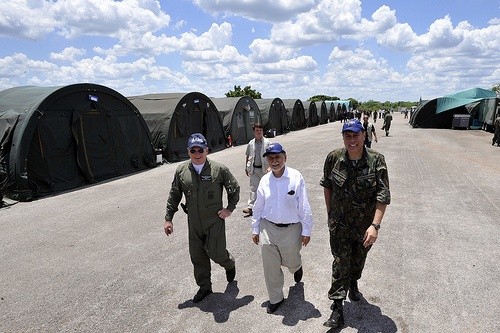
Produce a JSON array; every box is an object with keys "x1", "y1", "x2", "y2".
[
  {"x1": 409, "y1": 87, "x2": 500, "y2": 133},
  {"x1": 332, "y1": 100, "x2": 352, "y2": 110},
  {"x1": 254, "y1": 98, "x2": 328, "y2": 136},
  {"x1": 126, "y1": 92, "x2": 229, "y2": 166},
  {"x1": 209, "y1": 96, "x2": 264, "y2": 147},
  {"x1": 333, "y1": 102, "x2": 341, "y2": 121},
  {"x1": 325, "y1": 101, "x2": 335, "y2": 122},
  {"x1": 340, "y1": 102, "x2": 347, "y2": 112},
  {"x1": 0, "y1": 83, "x2": 159, "y2": 202}
]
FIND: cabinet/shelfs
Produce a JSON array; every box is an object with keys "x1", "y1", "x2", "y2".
[{"x1": 452, "y1": 114, "x2": 470, "y2": 130}]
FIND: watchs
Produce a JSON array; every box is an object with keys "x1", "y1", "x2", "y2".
[{"x1": 371, "y1": 223, "x2": 380, "y2": 231}]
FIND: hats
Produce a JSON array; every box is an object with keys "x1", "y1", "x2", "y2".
[
  {"x1": 263, "y1": 143, "x2": 286, "y2": 157},
  {"x1": 341, "y1": 119, "x2": 362, "y2": 132},
  {"x1": 188, "y1": 133, "x2": 207, "y2": 149}
]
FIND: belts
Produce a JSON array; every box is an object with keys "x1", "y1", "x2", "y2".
[{"x1": 270, "y1": 221, "x2": 295, "y2": 227}]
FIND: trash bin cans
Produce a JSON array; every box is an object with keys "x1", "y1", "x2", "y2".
[
  {"x1": 155, "y1": 149, "x2": 162, "y2": 165},
  {"x1": 274, "y1": 128, "x2": 276, "y2": 136}
]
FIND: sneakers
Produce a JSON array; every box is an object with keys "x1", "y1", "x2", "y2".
[
  {"x1": 323, "y1": 307, "x2": 344, "y2": 327},
  {"x1": 348, "y1": 280, "x2": 362, "y2": 301}
]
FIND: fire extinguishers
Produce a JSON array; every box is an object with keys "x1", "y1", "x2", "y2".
[{"x1": 228, "y1": 134, "x2": 231, "y2": 147}]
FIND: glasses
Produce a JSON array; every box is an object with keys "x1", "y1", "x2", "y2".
[{"x1": 189, "y1": 149, "x2": 204, "y2": 153}]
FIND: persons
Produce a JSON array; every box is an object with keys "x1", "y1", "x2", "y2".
[
  {"x1": 251, "y1": 142, "x2": 313, "y2": 314},
  {"x1": 404, "y1": 107, "x2": 413, "y2": 119},
  {"x1": 243, "y1": 123, "x2": 271, "y2": 215},
  {"x1": 373, "y1": 108, "x2": 390, "y2": 123},
  {"x1": 320, "y1": 118, "x2": 391, "y2": 328},
  {"x1": 339, "y1": 108, "x2": 372, "y2": 123},
  {"x1": 163, "y1": 133, "x2": 240, "y2": 304},
  {"x1": 362, "y1": 115, "x2": 378, "y2": 148},
  {"x1": 491, "y1": 112, "x2": 500, "y2": 146},
  {"x1": 381, "y1": 111, "x2": 392, "y2": 136}
]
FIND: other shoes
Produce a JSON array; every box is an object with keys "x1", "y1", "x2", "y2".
[
  {"x1": 192, "y1": 286, "x2": 212, "y2": 303},
  {"x1": 243, "y1": 209, "x2": 253, "y2": 216},
  {"x1": 267, "y1": 297, "x2": 284, "y2": 314},
  {"x1": 294, "y1": 266, "x2": 303, "y2": 283},
  {"x1": 226, "y1": 266, "x2": 235, "y2": 282}
]
